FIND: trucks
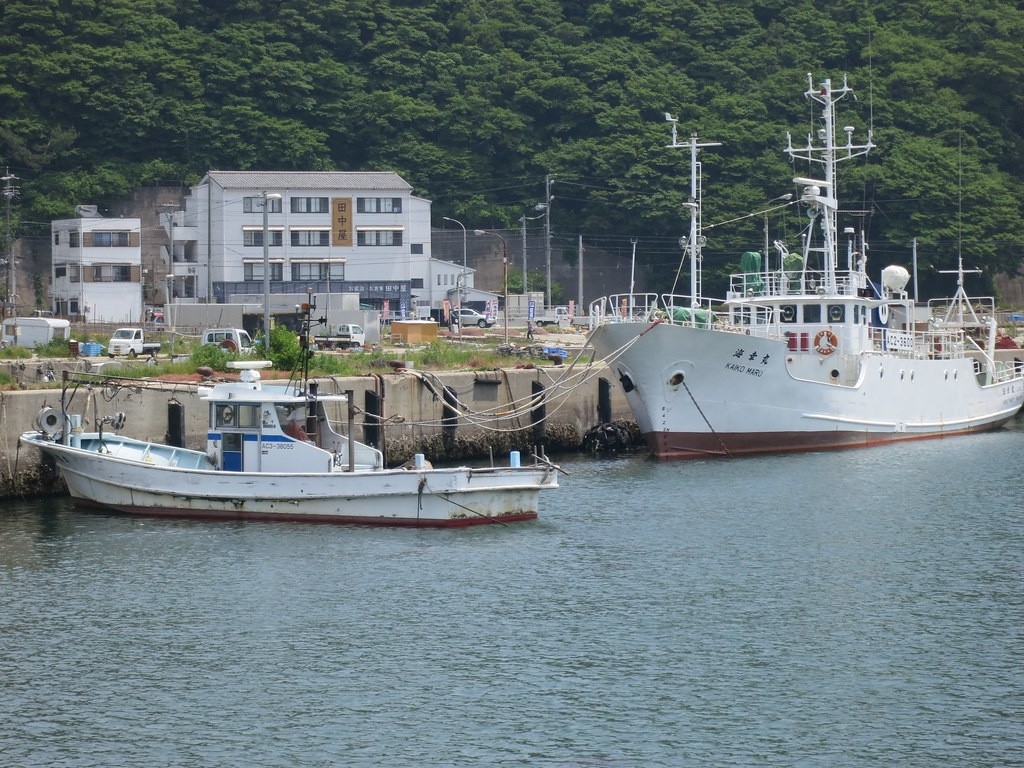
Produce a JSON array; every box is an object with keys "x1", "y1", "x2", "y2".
[
  {"x1": 533, "y1": 305, "x2": 574, "y2": 327},
  {"x1": 6, "y1": 309, "x2": 57, "y2": 318},
  {"x1": 108, "y1": 327, "x2": 162, "y2": 361},
  {"x1": 0, "y1": 318, "x2": 71, "y2": 351},
  {"x1": 313, "y1": 324, "x2": 366, "y2": 352},
  {"x1": 170, "y1": 329, "x2": 259, "y2": 366}
]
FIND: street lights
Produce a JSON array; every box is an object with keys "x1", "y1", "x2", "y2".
[
  {"x1": 761, "y1": 192, "x2": 794, "y2": 295},
  {"x1": 164, "y1": 273, "x2": 175, "y2": 329},
  {"x1": 262, "y1": 191, "x2": 282, "y2": 352},
  {"x1": 474, "y1": 228, "x2": 509, "y2": 343},
  {"x1": 442, "y1": 214, "x2": 468, "y2": 286}
]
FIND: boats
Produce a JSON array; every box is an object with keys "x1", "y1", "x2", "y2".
[
  {"x1": 17, "y1": 287, "x2": 561, "y2": 531},
  {"x1": 580, "y1": 16, "x2": 1024, "y2": 460}
]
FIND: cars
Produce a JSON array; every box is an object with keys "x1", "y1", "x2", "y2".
[
  {"x1": 146, "y1": 307, "x2": 164, "y2": 324},
  {"x1": 451, "y1": 307, "x2": 499, "y2": 329}
]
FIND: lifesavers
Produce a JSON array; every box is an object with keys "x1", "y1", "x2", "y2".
[{"x1": 814, "y1": 330, "x2": 838, "y2": 355}]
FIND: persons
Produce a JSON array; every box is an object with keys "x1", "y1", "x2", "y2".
[{"x1": 526, "y1": 320, "x2": 534, "y2": 340}]
